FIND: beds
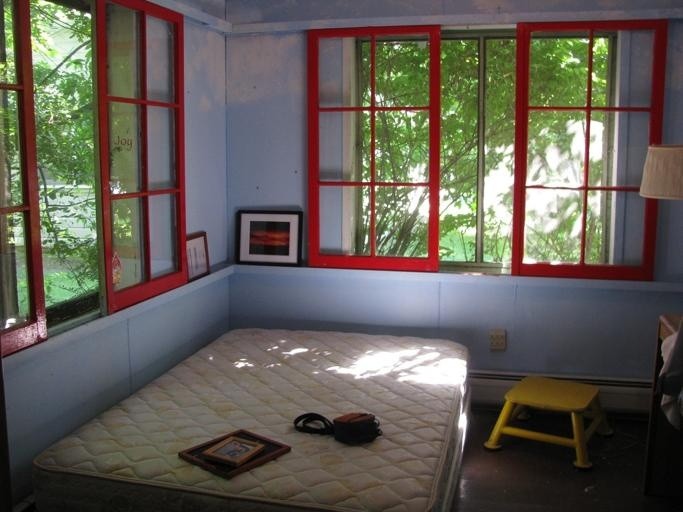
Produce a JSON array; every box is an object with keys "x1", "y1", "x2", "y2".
[{"x1": 29, "y1": 324, "x2": 474, "y2": 512}]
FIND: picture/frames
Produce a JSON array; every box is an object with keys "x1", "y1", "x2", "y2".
[
  {"x1": 185, "y1": 231, "x2": 211, "y2": 280},
  {"x1": 234, "y1": 209, "x2": 303, "y2": 266}
]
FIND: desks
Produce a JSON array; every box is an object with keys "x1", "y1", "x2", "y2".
[{"x1": 642, "y1": 310, "x2": 683, "y2": 506}]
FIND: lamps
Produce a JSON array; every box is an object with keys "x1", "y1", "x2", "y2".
[{"x1": 638, "y1": 145, "x2": 681, "y2": 202}]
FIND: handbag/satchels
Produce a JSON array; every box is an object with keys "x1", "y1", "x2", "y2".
[{"x1": 294, "y1": 411, "x2": 381, "y2": 445}]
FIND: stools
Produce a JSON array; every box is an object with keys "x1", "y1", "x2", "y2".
[{"x1": 482, "y1": 374, "x2": 615, "y2": 471}]
